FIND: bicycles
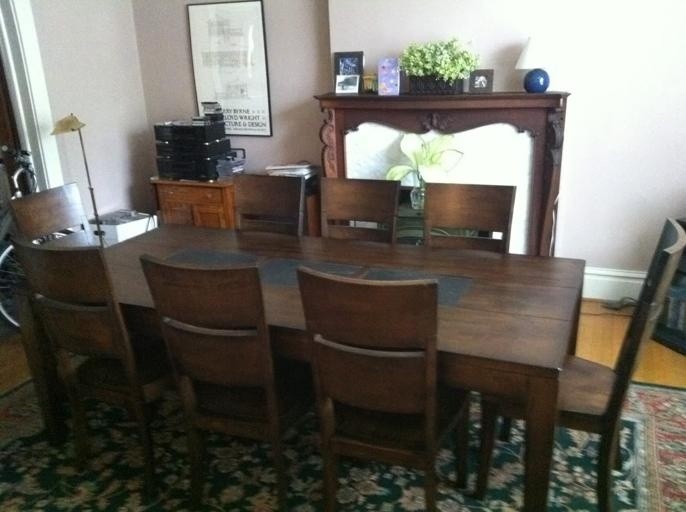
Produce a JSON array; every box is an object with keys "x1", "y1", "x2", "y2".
[{"x1": 0, "y1": 144, "x2": 82, "y2": 334}]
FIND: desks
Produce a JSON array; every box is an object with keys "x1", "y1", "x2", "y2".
[
  {"x1": 150, "y1": 170, "x2": 238, "y2": 229},
  {"x1": 104, "y1": 227, "x2": 586, "y2": 512},
  {"x1": 312, "y1": 90, "x2": 571, "y2": 259}
]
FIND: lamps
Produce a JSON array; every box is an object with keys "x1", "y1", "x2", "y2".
[
  {"x1": 515, "y1": 39, "x2": 558, "y2": 94},
  {"x1": 49, "y1": 110, "x2": 104, "y2": 250}
]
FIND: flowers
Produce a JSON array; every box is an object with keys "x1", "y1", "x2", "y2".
[
  {"x1": 383, "y1": 129, "x2": 468, "y2": 184},
  {"x1": 395, "y1": 36, "x2": 484, "y2": 89}
]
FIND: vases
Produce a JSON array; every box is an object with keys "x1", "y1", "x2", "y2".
[{"x1": 397, "y1": 67, "x2": 467, "y2": 95}]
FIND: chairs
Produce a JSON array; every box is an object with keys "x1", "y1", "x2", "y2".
[
  {"x1": 233, "y1": 172, "x2": 305, "y2": 239},
  {"x1": 10, "y1": 181, "x2": 99, "y2": 250},
  {"x1": 139, "y1": 254, "x2": 307, "y2": 508},
  {"x1": 296, "y1": 265, "x2": 468, "y2": 512},
  {"x1": 12, "y1": 241, "x2": 169, "y2": 497},
  {"x1": 320, "y1": 179, "x2": 403, "y2": 247},
  {"x1": 473, "y1": 218, "x2": 686, "y2": 512},
  {"x1": 425, "y1": 182, "x2": 517, "y2": 255}
]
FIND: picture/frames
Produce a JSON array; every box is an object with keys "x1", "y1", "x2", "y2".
[
  {"x1": 184, "y1": 1, "x2": 272, "y2": 139},
  {"x1": 468, "y1": 68, "x2": 495, "y2": 93},
  {"x1": 333, "y1": 50, "x2": 364, "y2": 95}
]
